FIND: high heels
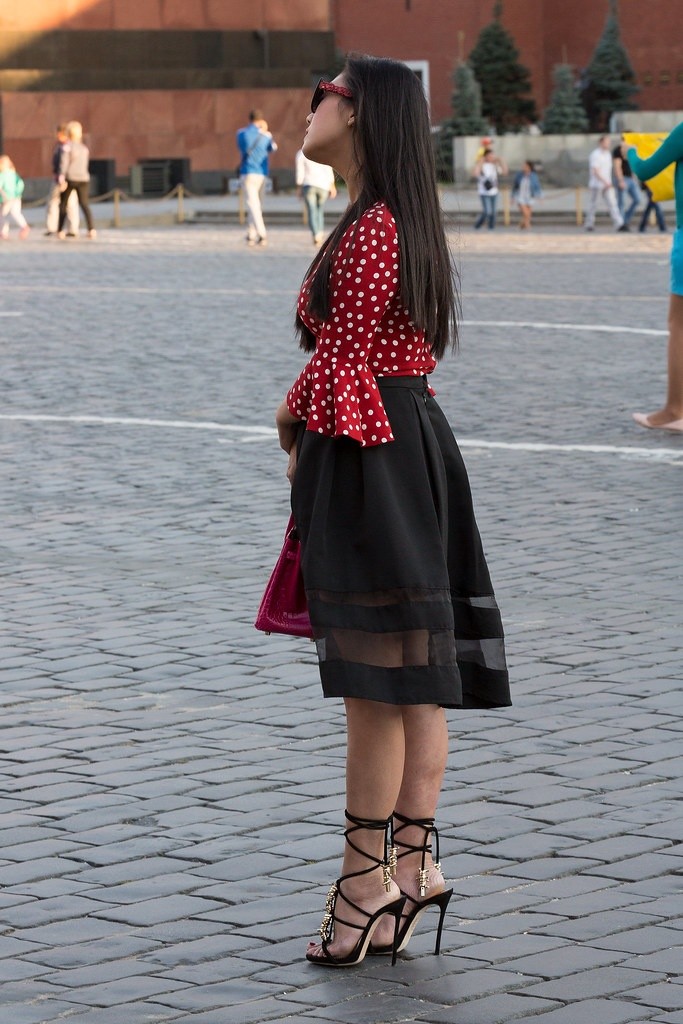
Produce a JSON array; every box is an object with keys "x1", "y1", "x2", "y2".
[
  {"x1": 366, "y1": 810, "x2": 452, "y2": 956},
  {"x1": 307, "y1": 809, "x2": 407, "y2": 968}
]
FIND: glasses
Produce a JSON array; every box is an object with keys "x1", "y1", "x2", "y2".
[{"x1": 311, "y1": 76, "x2": 353, "y2": 112}]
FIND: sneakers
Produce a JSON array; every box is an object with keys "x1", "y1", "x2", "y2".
[{"x1": 255, "y1": 424, "x2": 316, "y2": 641}]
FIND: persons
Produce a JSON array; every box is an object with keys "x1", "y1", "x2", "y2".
[
  {"x1": 42, "y1": 121, "x2": 98, "y2": 240},
  {"x1": 511, "y1": 160, "x2": 544, "y2": 231},
  {"x1": 585, "y1": 130, "x2": 670, "y2": 233},
  {"x1": 0, "y1": 155, "x2": 31, "y2": 239},
  {"x1": 621, "y1": 120, "x2": 683, "y2": 436},
  {"x1": 295, "y1": 144, "x2": 338, "y2": 245},
  {"x1": 236, "y1": 110, "x2": 278, "y2": 245},
  {"x1": 473, "y1": 137, "x2": 507, "y2": 231},
  {"x1": 275, "y1": 45, "x2": 479, "y2": 968}
]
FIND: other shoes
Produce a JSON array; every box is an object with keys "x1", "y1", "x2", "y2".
[
  {"x1": 87, "y1": 232, "x2": 95, "y2": 240},
  {"x1": 258, "y1": 238, "x2": 267, "y2": 245},
  {"x1": 245, "y1": 236, "x2": 254, "y2": 245},
  {"x1": 632, "y1": 411, "x2": 683, "y2": 431},
  {"x1": 19, "y1": 225, "x2": 29, "y2": 240},
  {"x1": 44, "y1": 232, "x2": 55, "y2": 236},
  {"x1": 66, "y1": 232, "x2": 76, "y2": 237},
  {"x1": 57, "y1": 231, "x2": 65, "y2": 239}
]
